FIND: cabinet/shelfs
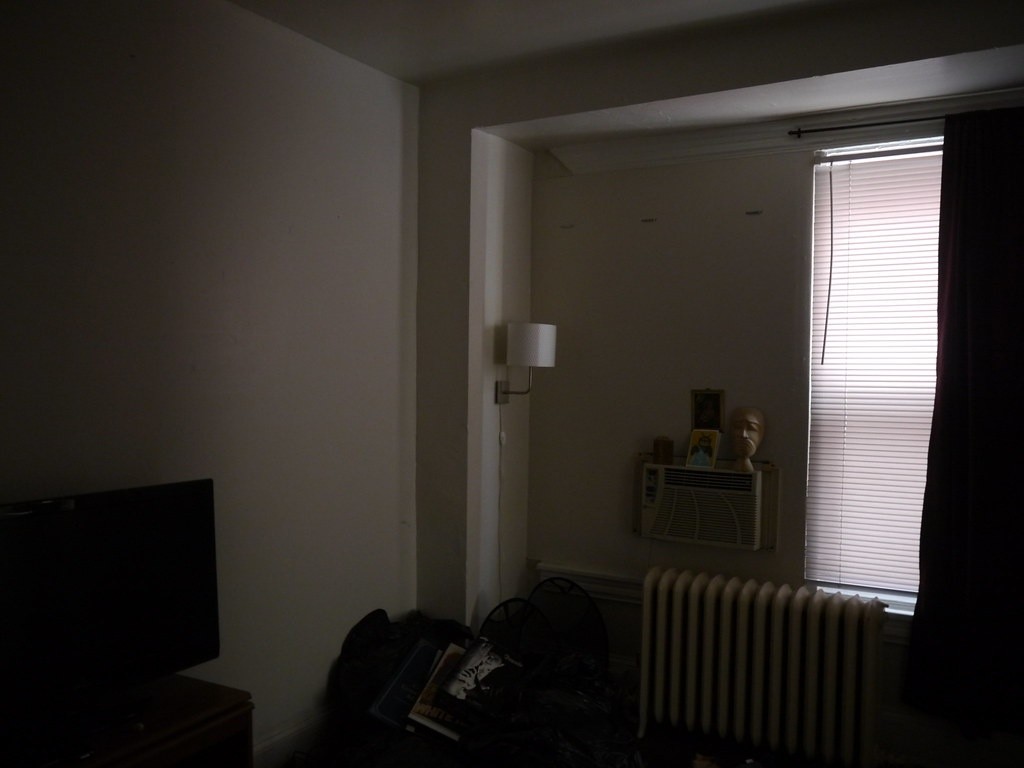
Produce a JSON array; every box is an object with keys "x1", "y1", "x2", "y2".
[{"x1": 1, "y1": 674, "x2": 255, "y2": 768}]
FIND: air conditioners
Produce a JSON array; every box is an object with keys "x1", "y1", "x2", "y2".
[{"x1": 637, "y1": 462, "x2": 770, "y2": 552}]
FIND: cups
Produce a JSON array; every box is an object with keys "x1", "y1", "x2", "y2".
[{"x1": 653, "y1": 436, "x2": 674, "y2": 465}]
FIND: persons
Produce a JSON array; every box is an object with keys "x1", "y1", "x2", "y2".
[
  {"x1": 728, "y1": 407, "x2": 765, "y2": 472},
  {"x1": 435, "y1": 644, "x2": 506, "y2": 723}
]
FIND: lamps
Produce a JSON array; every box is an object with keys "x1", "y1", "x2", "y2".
[{"x1": 495, "y1": 322, "x2": 557, "y2": 404}]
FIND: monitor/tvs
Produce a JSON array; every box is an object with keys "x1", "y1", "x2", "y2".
[{"x1": 0, "y1": 479, "x2": 219, "y2": 746}]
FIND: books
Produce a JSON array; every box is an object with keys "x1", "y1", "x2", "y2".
[{"x1": 368, "y1": 633, "x2": 522, "y2": 743}]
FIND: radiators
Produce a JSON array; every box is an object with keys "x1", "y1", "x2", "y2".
[{"x1": 636, "y1": 562, "x2": 889, "y2": 768}]
